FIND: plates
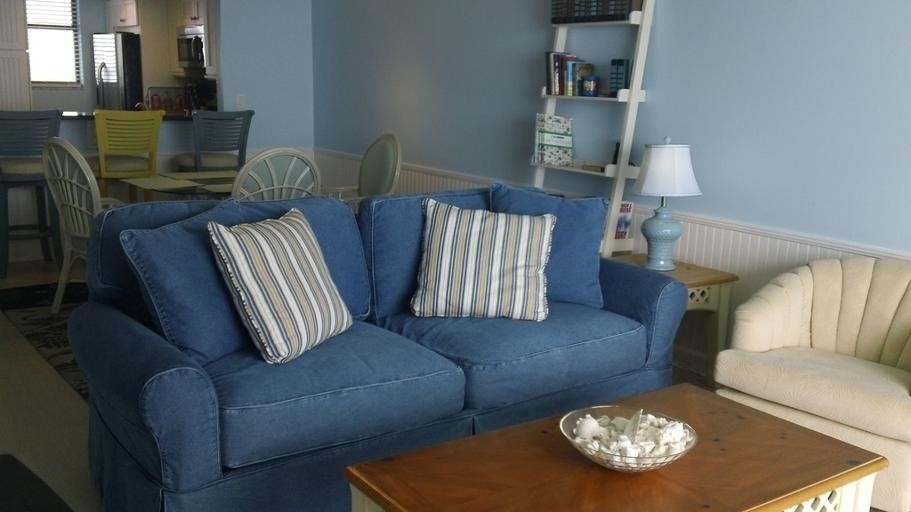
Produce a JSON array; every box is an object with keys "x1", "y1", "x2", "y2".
[{"x1": 559, "y1": 405, "x2": 698, "y2": 473}]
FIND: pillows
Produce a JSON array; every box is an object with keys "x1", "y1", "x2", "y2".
[
  {"x1": 207, "y1": 207, "x2": 355, "y2": 365},
  {"x1": 410, "y1": 192, "x2": 557, "y2": 325},
  {"x1": 117, "y1": 196, "x2": 252, "y2": 371},
  {"x1": 488, "y1": 178, "x2": 609, "y2": 310}
]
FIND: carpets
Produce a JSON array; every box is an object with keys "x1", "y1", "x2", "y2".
[{"x1": 6, "y1": 303, "x2": 92, "y2": 403}]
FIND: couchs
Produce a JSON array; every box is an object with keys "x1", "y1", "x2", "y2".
[{"x1": 65, "y1": 182, "x2": 686, "y2": 512}]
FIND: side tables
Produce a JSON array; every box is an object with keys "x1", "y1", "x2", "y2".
[{"x1": 617, "y1": 252, "x2": 737, "y2": 387}]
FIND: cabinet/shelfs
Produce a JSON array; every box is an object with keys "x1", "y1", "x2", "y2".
[
  {"x1": 532, "y1": 2, "x2": 655, "y2": 257},
  {"x1": 107, "y1": 1, "x2": 138, "y2": 27},
  {"x1": 177, "y1": 1, "x2": 204, "y2": 27}
]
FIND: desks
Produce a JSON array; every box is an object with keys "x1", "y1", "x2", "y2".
[{"x1": 125, "y1": 170, "x2": 240, "y2": 203}]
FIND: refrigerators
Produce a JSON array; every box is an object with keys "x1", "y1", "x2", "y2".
[{"x1": 92, "y1": 31, "x2": 143, "y2": 111}]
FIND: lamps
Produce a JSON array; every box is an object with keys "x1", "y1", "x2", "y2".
[{"x1": 632, "y1": 136, "x2": 706, "y2": 271}]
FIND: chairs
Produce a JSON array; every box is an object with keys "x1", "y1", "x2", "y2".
[
  {"x1": 43, "y1": 135, "x2": 130, "y2": 316},
  {"x1": 84, "y1": 109, "x2": 164, "y2": 196},
  {"x1": 230, "y1": 147, "x2": 327, "y2": 203},
  {"x1": 1, "y1": 109, "x2": 65, "y2": 274},
  {"x1": 325, "y1": 134, "x2": 402, "y2": 211},
  {"x1": 713, "y1": 256, "x2": 908, "y2": 512},
  {"x1": 181, "y1": 108, "x2": 257, "y2": 171}
]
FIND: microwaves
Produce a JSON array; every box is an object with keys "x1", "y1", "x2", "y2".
[{"x1": 177, "y1": 27, "x2": 204, "y2": 68}]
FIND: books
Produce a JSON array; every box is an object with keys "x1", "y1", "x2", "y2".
[
  {"x1": 609, "y1": 59, "x2": 633, "y2": 97},
  {"x1": 615, "y1": 201, "x2": 633, "y2": 242},
  {"x1": 534, "y1": 112, "x2": 573, "y2": 167},
  {"x1": 544, "y1": 50, "x2": 594, "y2": 99}
]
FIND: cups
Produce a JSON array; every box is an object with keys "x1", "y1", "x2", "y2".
[{"x1": 582, "y1": 74, "x2": 600, "y2": 97}]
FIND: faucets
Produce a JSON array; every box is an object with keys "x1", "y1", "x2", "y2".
[{"x1": 135, "y1": 101, "x2": 147, "y2": 110}]
